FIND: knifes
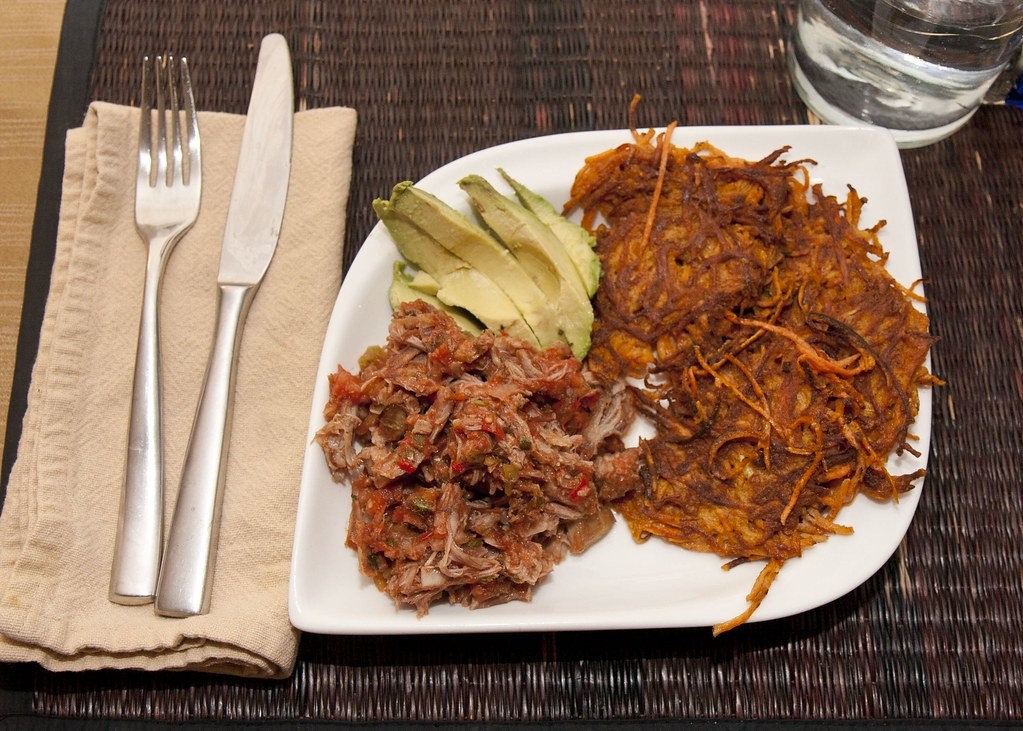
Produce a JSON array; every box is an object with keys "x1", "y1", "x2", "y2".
[{"x1": 156, "y1": 33, "x2": 295, "y2": 620}]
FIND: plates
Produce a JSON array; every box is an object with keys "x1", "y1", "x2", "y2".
[{"x1": 290, "y1": 126, "x2": 933, "y2": 634}]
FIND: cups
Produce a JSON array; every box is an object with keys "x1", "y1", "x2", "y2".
[{"x1": 785, "y1": 0, "x2": 1022, "y2": 154}]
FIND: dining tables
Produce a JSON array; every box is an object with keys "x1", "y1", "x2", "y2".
[{"x1": 1, "y1": 1, "x2": 1023, "y2": 730}]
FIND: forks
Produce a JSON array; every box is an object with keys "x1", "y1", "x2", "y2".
[{"x1": 107, "y1": 54, "x2": 204, "y2": 606}]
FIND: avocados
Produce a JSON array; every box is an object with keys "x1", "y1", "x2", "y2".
[{"x1": 369, "y1": 165, "x2": 605, "y2": 364}]
